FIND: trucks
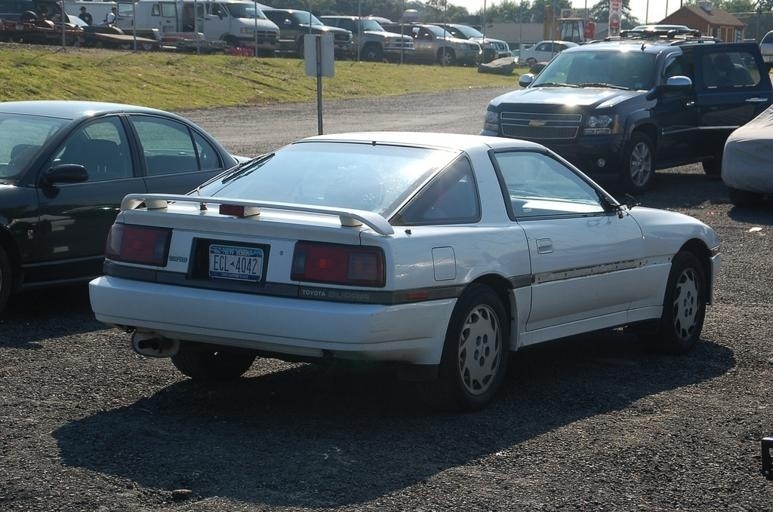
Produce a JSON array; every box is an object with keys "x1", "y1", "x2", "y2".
[{"x1": 115, "y1": 0, "x2": 280, "y2": 58}]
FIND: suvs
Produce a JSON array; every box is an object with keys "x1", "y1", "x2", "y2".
[{"x1": 263, "y1": 8, "x2": 511, "y2": 67}]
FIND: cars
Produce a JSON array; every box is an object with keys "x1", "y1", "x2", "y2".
[
  {"x1": 478, "y1": 28, "x2": 772, "y2": 195},
  {"x1": 720, "y1": 103, "x2": 772, "y2": 208},
  {"x1": 1, "y1": 99, "x2": 253, "y2": 314},
  {"x1": 757, "y1": 29, "x2": 772, "y2": 64},
  {"x1": 88, "y1": 129, "x2": 721, "y2": 413},
  {"x1": 519, "y1": 40, "x2": 579, "y2": 66}
]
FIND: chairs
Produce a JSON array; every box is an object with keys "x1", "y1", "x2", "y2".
[{"x1": 10, "y1": 139, "x2": 119, "y2": 174}]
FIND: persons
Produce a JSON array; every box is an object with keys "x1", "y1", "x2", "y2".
[
  {"x1": 713, "y1": 54, "x2": 755, "y2": 86},
  {"x1": 101, "y1": 6, "x2": 116, "y2": 27},
  {"x1": 78, "y1": 7, "x2": 92, "y2": 26}
]
